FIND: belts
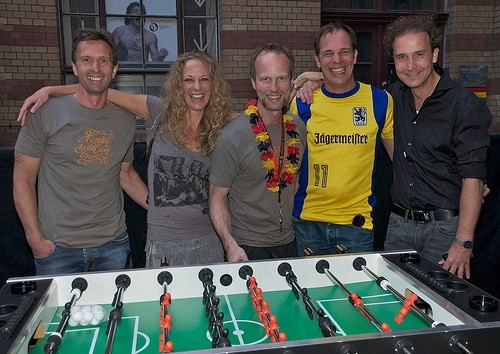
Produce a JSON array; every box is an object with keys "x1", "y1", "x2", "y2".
[{"x1": 390, "y1": 202, "x2": 460, "y2": 224}]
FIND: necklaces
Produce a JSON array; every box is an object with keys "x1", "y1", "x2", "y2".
[{"x1": 248, "y1": 98, "x2": 302, "y2": 191}]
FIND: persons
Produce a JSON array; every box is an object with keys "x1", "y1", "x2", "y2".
[
  {"x1": 381, "y1": 14, "x2": 493, "y2": 280},
  {"x1": 111, "y1": 1, "x2": 169, "y2": 62},
  {"x1": 13, "y1": 26, "x2": 151, "y2": 276},
  {"x1": 208, "y1": 41, "x2": 307, "y2": 263},
  {"x1": 283, "y1": 21, "x2": 491, "y2": 258},
  {"x1": 16, "y1": 49, "x2": 327, "y2": 269}
]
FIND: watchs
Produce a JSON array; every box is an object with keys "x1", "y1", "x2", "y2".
[{"x1": 452, "y1": 237, "x2": 475, "y2": 249}]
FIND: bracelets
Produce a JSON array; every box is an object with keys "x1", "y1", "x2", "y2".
[{"x1": 291, "y1": 73, "x2": 306, "y2": 91}]
